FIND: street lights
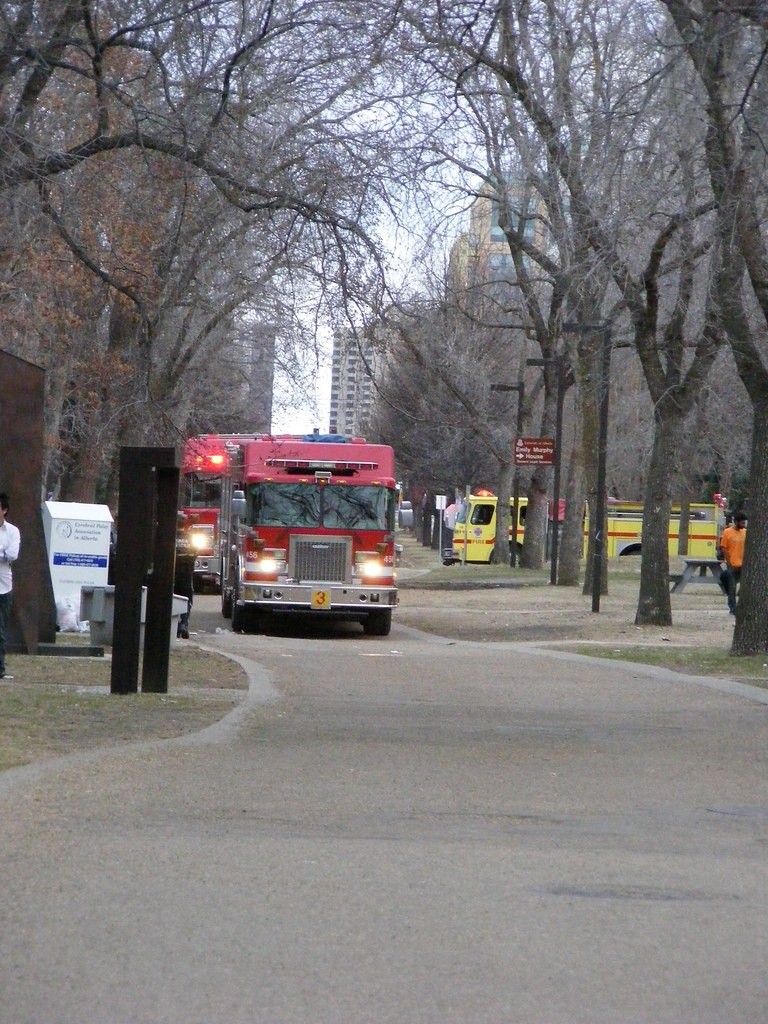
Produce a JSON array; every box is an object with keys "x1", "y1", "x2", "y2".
[
  {"x1": 526, "y1": 357, "x2": 566, "y2": 589},
  {"x1": 490, "y1": 382, "x2": 524, "y2": 568},
  {"x1": 562, "y1": 320, "x2": 614, "y2": 613}
]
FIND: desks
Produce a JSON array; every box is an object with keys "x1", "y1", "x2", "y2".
[{"x1": 672, "y1": 559, "x2": 728, "y2": 596}]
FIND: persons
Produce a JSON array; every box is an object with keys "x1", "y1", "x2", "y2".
[
  {"x1": 715, "y1": 513, "x2": 747, "y2": 626},
  {"x1": 172, "y1": 529, "x2": 197, "y2": 638},
  {"x1": 0, "y1": 493, "x2": 21, "y2": 680}
]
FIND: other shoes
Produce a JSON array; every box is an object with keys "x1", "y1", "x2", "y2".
[
  {"x1": 730, "y1": 609, "x2": 734, "y2": 615},
  {"x1": 179, "y1": 624, "x2": 189, "y2": 639},
  {"x1": 0, "y1": 674, "x2": 14, "y2": 681}
]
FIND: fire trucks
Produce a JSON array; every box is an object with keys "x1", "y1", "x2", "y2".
[
  {"x1": 174, "y1": 428, "x2": 270, "y2": 598},
  {"x1": 449, "y1": 489, "x2": 730, "y2": 569},
  {"x1": 222, "y1": 432, "x2": 400, "y2": 637}
]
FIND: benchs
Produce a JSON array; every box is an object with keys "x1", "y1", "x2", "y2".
[{"x1": 668, "y1": 572, "x2": 683, "y2": 578}]
